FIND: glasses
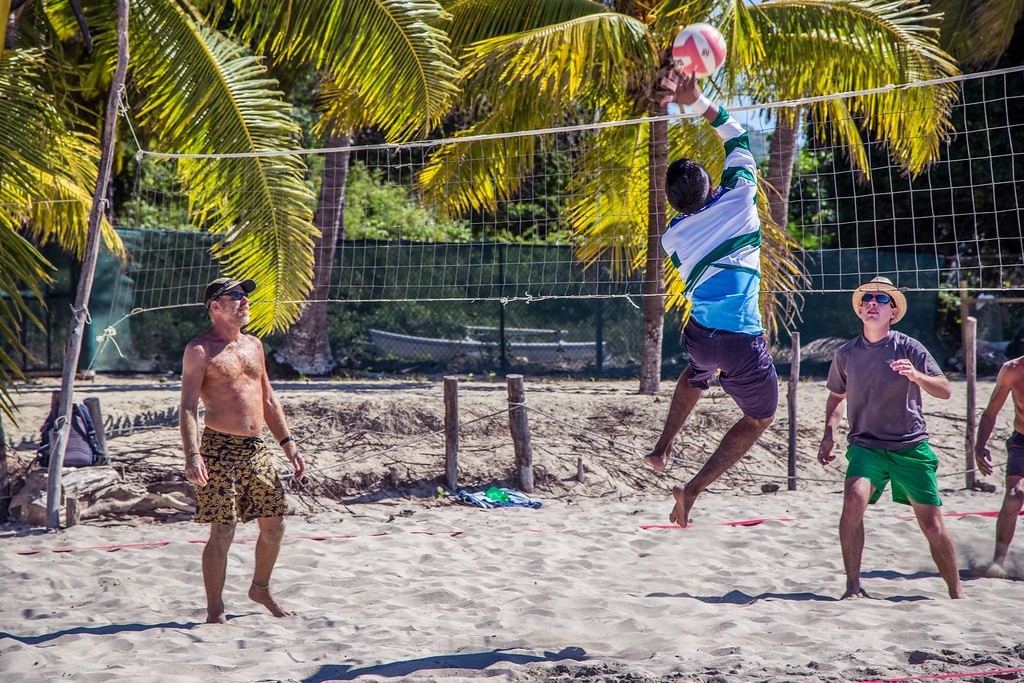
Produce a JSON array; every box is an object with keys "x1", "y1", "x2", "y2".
[
  {"x1": 861, "y1": 294, "x2": 896, "y2": 307},
  {"x1": 218, "y1": 291, "x2": 248, "y2": 301}
]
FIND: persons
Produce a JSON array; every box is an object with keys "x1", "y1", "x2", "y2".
[
  {"x1": 973, "y1": 356, "x2": 1024, "y2": 567},
  {"x1": 817, "y1": 276, "x2": 968, "y2": 599},
  {"x1": 642, "y1": 66, "x2": 778, "y2": 529},
  {"x1": 179, "y1": 277, "x2": 305, "y2": 624}
]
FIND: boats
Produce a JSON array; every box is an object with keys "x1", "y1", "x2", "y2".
[{"x1": 366, "y1": 328, "x2": 608, "y2": 366}]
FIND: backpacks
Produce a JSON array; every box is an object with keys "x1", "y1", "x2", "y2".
[{"x1": 38, "y1": 402, "x2": 105, "y2": 466}]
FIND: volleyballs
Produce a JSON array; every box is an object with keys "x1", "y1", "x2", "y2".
[{"x1": 672, "y1": 21, "x2": 728, "y2": 79}]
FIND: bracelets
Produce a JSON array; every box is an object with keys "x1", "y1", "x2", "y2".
[
  {"x1": 186, "y1": 452, "x2": 200, "y2": 461},
  {"x1": 280, "y1": 436, "x2": 294, "y2": 446},
  {"x1": 689, "y1": 95, "x2": 710, "y2": 116}
]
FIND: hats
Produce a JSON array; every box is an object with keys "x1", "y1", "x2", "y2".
[
  {"x1": 852, "y1": 277, "x2": 908, "y2": 325},
  {"x1": 204, "y1": 277, "x2": 256, "y2": 312}
]
